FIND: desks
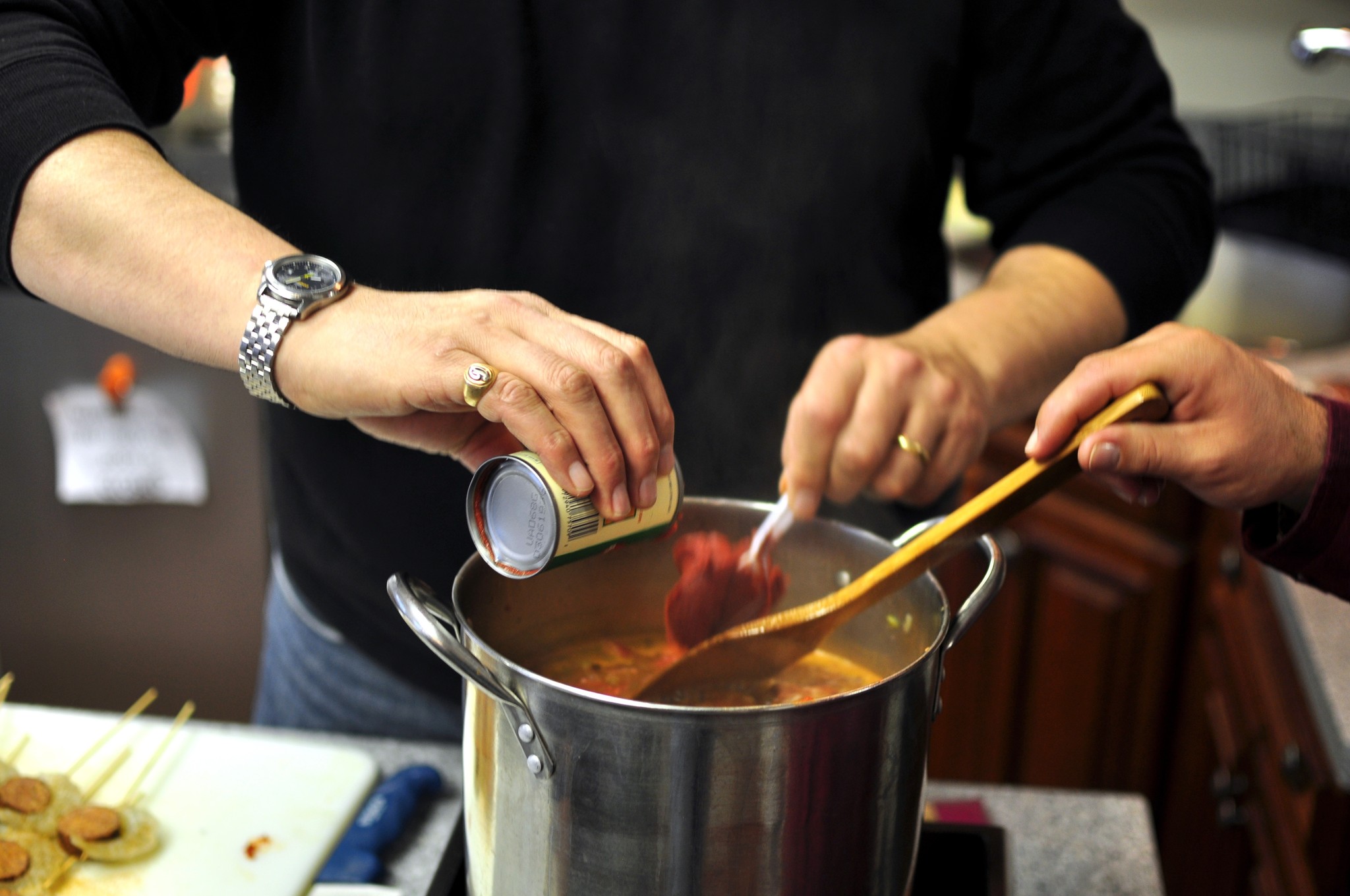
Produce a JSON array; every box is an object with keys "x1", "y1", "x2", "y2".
[{"x1": 2, "y1": 699, "x2": 1166, "y2": 896}]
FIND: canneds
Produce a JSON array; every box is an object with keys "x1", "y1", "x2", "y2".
[{"x1": 464, "y1": 447, "x2": 685, "y2": 581}]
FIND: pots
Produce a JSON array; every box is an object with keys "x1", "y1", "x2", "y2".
[{"x1": 388, "y1": 496, "x2": 1005, "y2": 896}]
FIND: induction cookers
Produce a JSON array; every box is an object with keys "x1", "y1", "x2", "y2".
[{"x1": 429, "y1": 812, "x2": 1001, "y2": 896}]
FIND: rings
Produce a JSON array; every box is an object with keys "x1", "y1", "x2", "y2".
[
  {"x1": 896, "y1": 432, "x2": 933, "y2": 466},
  {"x1": 461, "y1": 362, "x2": 502, "y2": 410}
]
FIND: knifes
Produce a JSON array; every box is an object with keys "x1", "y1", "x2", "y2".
[{"x1": 307, "y1": 764, "x2": 443, "y2": 895}]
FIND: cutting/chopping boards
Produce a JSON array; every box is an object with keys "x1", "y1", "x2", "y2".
[{"x1": 0, "y1": 704, "x2": 383, "y2": 896}]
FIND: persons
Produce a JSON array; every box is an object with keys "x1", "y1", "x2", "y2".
[
  {"x1": 1020, "y1": 316, "x2": 1348, "y2": 611},
  {"x1": 2, "y1": 3, "x2": 1230, "y2": 742}
]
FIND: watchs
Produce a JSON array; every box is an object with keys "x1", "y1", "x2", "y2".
[{"x1": 236, "y1": 252, "x2": 364, "y2": 412}]
